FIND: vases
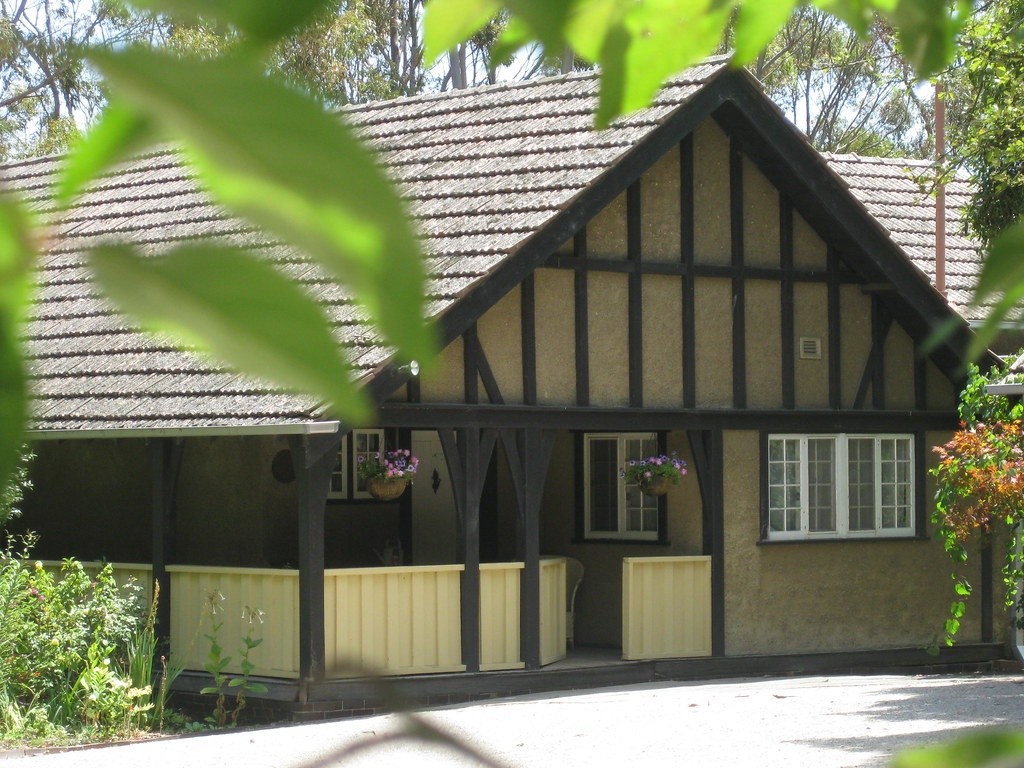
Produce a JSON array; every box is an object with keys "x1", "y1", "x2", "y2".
[{"x1": 641, "y1": 476, "x2": 674, "y2": 495}]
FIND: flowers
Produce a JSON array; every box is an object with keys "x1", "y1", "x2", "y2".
[
  {"x1": 620, "y1": 451, "x2": 688, "y2": 484},
  {"x1": 357, "y1": 447, "x2": 420, "y2": 487}
]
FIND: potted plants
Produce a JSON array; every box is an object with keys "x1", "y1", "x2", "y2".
[{"x1": 367, "y1": 476, "x2": 407, "y2": 499}]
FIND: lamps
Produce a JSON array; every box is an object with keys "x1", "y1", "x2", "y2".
[{"x1": 391, "y1": 359, "x2": 420, "y2": 378}]
